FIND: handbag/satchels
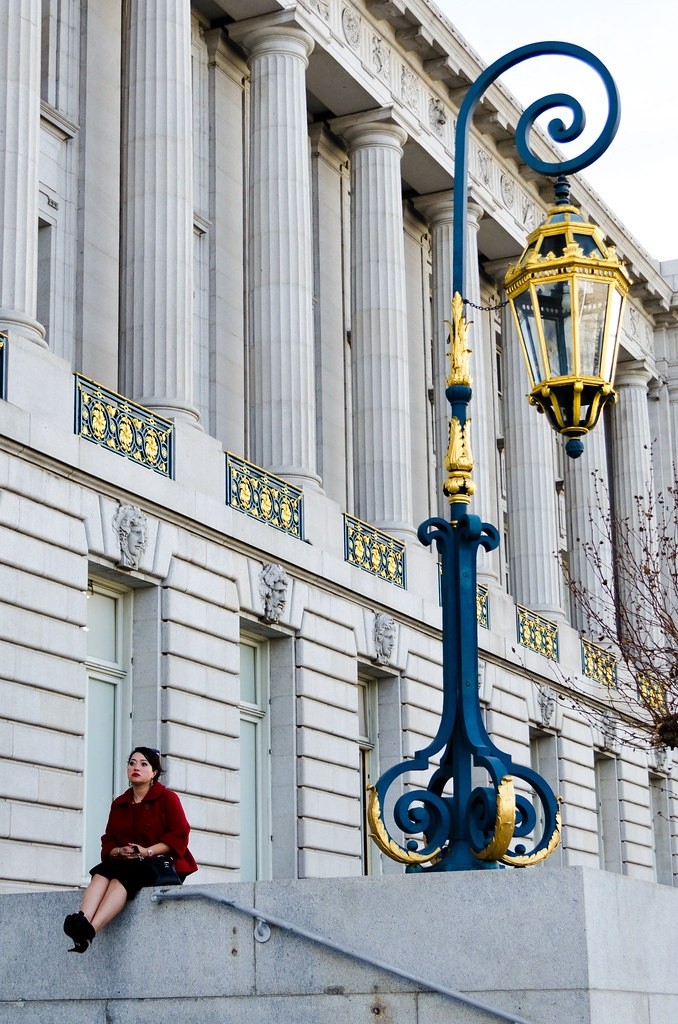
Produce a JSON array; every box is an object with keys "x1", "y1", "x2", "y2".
[{"x1": 150, "y1": 855, "x2": 183, "y2": 885}]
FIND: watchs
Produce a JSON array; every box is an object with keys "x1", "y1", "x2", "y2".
[{"x1": 147, "y1": 848, "x2": 152, "y2": 856}]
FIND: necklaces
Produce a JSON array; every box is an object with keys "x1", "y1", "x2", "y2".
[{"x1": 133, "y1": 800, "x2": 136, "y2": 804}]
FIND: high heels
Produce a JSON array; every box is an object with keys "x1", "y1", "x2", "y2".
[
  {"x1": 63, "y1": 915, "x2": 89, "y2": 953},
  {"x1": 72, "y1": 911, "x2": 96, "y2": 946}
]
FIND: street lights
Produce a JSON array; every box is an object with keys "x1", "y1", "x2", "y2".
[{"x1": 368, "y1": 32, "x2": 628, "y2": 873}]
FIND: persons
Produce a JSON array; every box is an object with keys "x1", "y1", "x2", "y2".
[
  {"x1": 263, "y1": 565, "x2": 289, "y2": 622},
  {"x1": 64, "y1": 747, "x2": 198, "y2": 953},
  {"x1": 538, "y1": 686, "x2": 555, "y2": 727},
  {"x1": 115, "y1": 505, "x2": 147, "y2": 568},
  {"x1": 374, "y1": 613, "x2": 397, "y2": 665},
  {"x1": 655, "y1": 737, "x2": 668, "y2": 772},
  {"x1": 479, "y1": 659, "x2": 485, "y2": 690},
  {"x1": 603, "y1": 711, "x2": 618, "y2": 750}
]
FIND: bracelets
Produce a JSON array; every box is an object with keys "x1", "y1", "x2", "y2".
[{"x1": 118, "y1": 848, "x2": 120, "y2": 853}]
